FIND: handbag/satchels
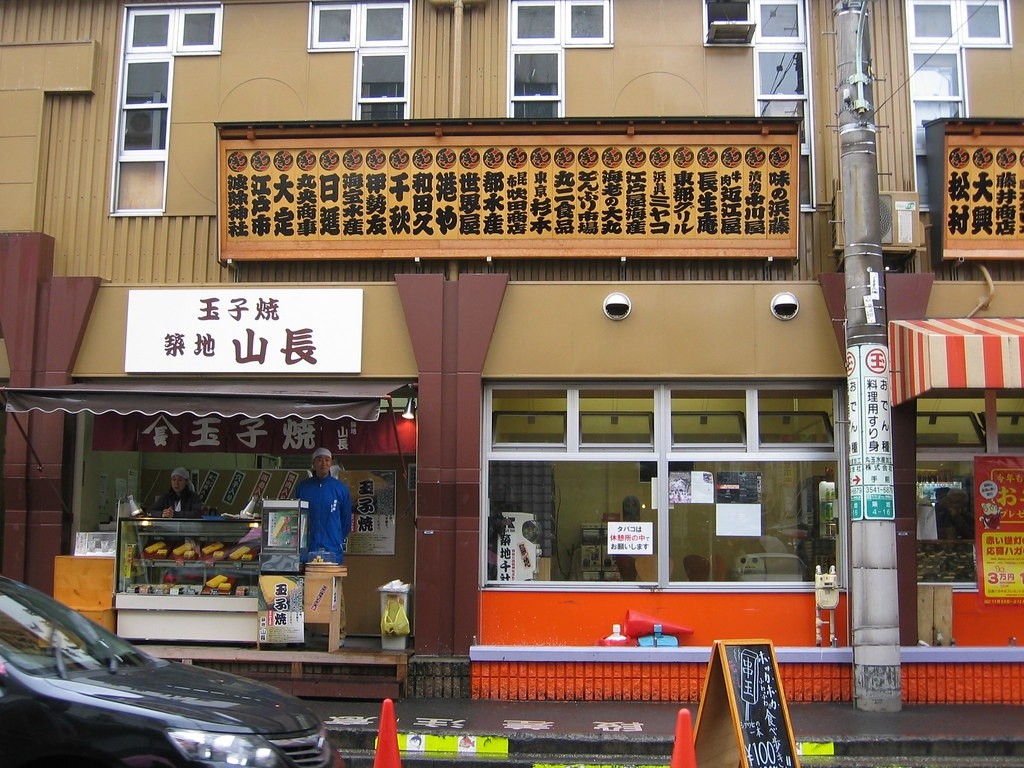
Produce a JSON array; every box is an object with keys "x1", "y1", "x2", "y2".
[{"x1": 380, "y1": 594, "x2": 410, "y2": 636}]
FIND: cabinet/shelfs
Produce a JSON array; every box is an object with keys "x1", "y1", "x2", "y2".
[{"x1": 116, "y1": 516, "x2": 263, "y2": 645}]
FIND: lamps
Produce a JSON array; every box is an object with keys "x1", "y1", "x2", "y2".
[{"x1": 401, "y1": 397, "x2": 415, "y2": 419}]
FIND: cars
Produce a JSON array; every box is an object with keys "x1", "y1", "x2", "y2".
[
  {"x1": 0, "y1": 576, "x2": 349, "y2": 768},
  {"x1": 725, "y1": 551, "x2": 814, "y2": 583}
]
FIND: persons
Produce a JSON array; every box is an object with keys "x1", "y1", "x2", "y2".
[
  {"x1": 295, "y1": 447, "x2": 351, "y2": 649},
  {"x1": 145, "y1": 466, "x2": 202, "y2": 559}
]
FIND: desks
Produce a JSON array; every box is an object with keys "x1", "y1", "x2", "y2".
[{"x1": 258, "y1": 571, "x2": 346, "y2": 653}]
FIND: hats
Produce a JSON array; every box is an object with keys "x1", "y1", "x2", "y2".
[
  {"x1": 171, "y1": 467, "x2": 188, "y2": 479},
  {"x1": 311, "y1": 447, "x2": 332, "y2": 464}
]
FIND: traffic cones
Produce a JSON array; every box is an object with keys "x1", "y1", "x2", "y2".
[
  {"x1": 671, "y1": 707, "x2": 700, "y2": 768},
  {"x1": 372, "y1": 697, "x2": 403, "y2": 768}
]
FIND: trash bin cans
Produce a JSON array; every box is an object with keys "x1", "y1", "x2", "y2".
[{"x1": 377, "y1": 582, "x2": 413, "y2": 650}]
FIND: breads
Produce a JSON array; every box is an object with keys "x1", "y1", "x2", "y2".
[
  {"x1": 205, "y1": 575, "x2": 231, "y2": 590},
  {"x1": 259, "y1": 575, "x2": 298, "y2": 606},
  {"x1": 144, "y1": 541, "x2": 253, "y2": 561}
]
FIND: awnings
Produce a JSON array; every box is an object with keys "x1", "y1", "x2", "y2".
[
  {"x1": 888, "y1": 318, "x2": 1024, "y2": 407},
  {"x1": 1, "y1": 379, "x2": 416, "y2": 525}
]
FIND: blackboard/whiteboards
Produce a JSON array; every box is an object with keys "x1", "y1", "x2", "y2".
[{"x1": 692, "y1": 638, "x2": 800, "y2": 768}]
